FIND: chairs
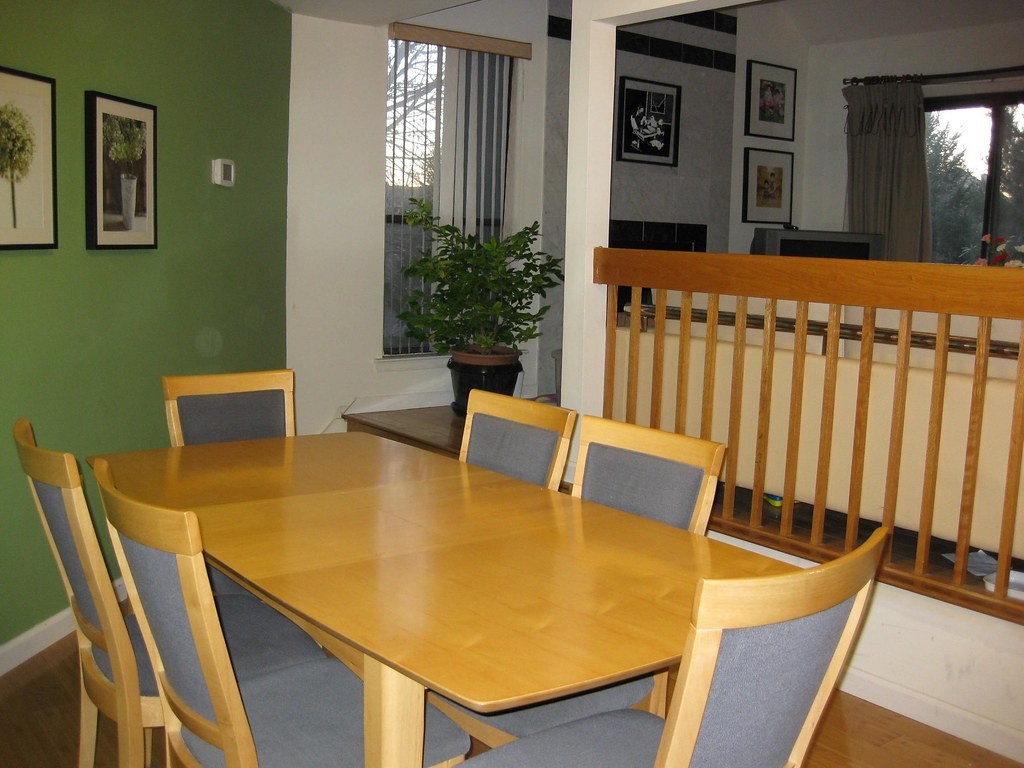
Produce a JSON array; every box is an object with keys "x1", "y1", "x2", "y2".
[
  {"x1": 428, "y1": 415, "x2": 726, "y2": 749},
  {"x1": 96, "y1": 459, "x2": 471, "y2": 768},
  {"x1": 458, "y1": 526, "x2": 892, "y2": 768},
  {"x1": 459, "y1": 390, "x2": 577, "y2": 491},
  {"x1": 13, "y1": 419, "x2": 326, "y2": 768},
  {"x1": 160, "y1": 369, "x2": 297, "y2": 602}
]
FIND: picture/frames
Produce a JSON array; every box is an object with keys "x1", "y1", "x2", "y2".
[
  {"x1": 84, "y1": 90, "x2": 158, "y2": 250},
  {"x1": 741, "y1": 147, "x2": 794, "y2": 224},
  {"x1": 744, "y1": 60, "x2": 798, "y2": 141},
  {"x1": 616, "y1": 75, "x2": 682, "y2": 167},
  {"x1": 0, "y1": 66, "x2": 58, "y2": 250}
]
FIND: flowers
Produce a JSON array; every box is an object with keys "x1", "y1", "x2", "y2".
[{"x1": 975, "y1": 234, "x2": 1024, "y2": 268}]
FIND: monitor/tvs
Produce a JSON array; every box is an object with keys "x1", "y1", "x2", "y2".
[{"x1": 749, "y1": 228, "x2": 884, "y2": 262}]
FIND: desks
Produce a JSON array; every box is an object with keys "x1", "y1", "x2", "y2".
[{"x1": 87, "y1": 429, "x2": 806, "y2": 768}]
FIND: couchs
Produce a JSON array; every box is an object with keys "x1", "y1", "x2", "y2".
[{"x1": 609, "y1": 289, "x2": 1024, "y2": 562}]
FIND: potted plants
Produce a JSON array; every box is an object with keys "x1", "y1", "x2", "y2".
[{"x1": 393, "y1": 199, "x2": 565, "y2": 415}]
